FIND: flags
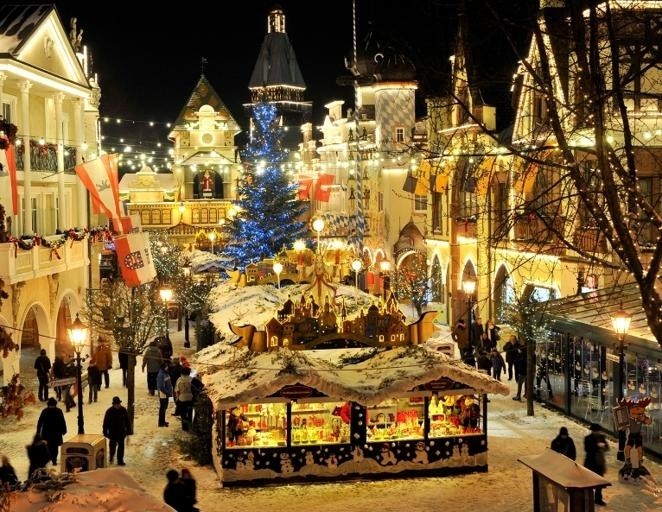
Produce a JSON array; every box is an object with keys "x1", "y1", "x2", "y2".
[
  {"x1": 505, "y1": 139, "x2": 556, "y2": 196},
  {"x1": 106, "y1": 215, "x2": 160, "y2": 290},
  {"x1": 312, "y1": 172, "x2": 335, "y2": 206},
  {"x1": 401, "y1": 149, "x2": 451, "y2": 198},
  {"x1": 293, "y1": 169, "x2": 316, "y2": 204},
  {"x1": 70, "y1": 149, "x2": 126, "y2": 221},
  {"x1": 458, "y1": 147, "x2": 499, "y2": 200},
  {"x1": 0, "y1": 131, "x2": 23, "y2": 221}
]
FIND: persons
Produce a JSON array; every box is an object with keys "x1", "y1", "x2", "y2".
[
  {"x1": 141, "y1": 329, "x2": 197, "y2": 433},
  {"x1": 28, "y1": 336, "x2": 136, "y2": 412},
  {"x1": 447, "y1": 314, "x2": 556, "y2": 404},
  {"x1": 549, "y1": 425, "x2": 579, "y2": 463},
  {"x1": 100, "y1": 395, "x2": 135, "y2": 467},
  {"x1": 180, "y1": 466, "x2": 199, "y2": 507},
  {"x1": 581, "y1": 422, "x2": 612, "y2": 507},
  {"x1": 158, "y1": 469, "x2": 204, "y2": 511},
  {"x1": 33, "y1": 396, "x2": 69, "y2": 466},
  {"x1": 0, "y1": 453, "x2": 19, "y2": 487},
  {"x1": 23, "y1": 434, "x2": 51, "y2": 481}
]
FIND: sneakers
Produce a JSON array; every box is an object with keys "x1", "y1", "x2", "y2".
[{"x1": 595, "y1": 499, "x2": 606, "y2": 506}]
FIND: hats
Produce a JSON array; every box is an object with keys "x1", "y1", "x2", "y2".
[
  {"x1": 47, "y1": 397, "x2": 57, "y2": 406},
  {"x1": 112, "y1": 397, "x2": 122, "y2": 404}
]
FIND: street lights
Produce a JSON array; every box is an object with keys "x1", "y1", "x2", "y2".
[
  {"x1": 273, "y1": 217, "x2": 394, "y2": 307},
  {"x1": 159, "y1": 285, "x2": 173, "y2": 337},
  {"x1": 461, "y1": 271, "x2": 478, "y2": 365},
  {"x1": 67, "y1": 311, "x2": 91, "y2": 434},
  {"x1": 208, "y1": 232, "x2": 216, "y2": 253},
  {"x1": 609, "y1": 299, "x2": 634, "y2": 462},
  {"x1": 182, "y1": 261, "x2": 192, "y2": 347}
]
